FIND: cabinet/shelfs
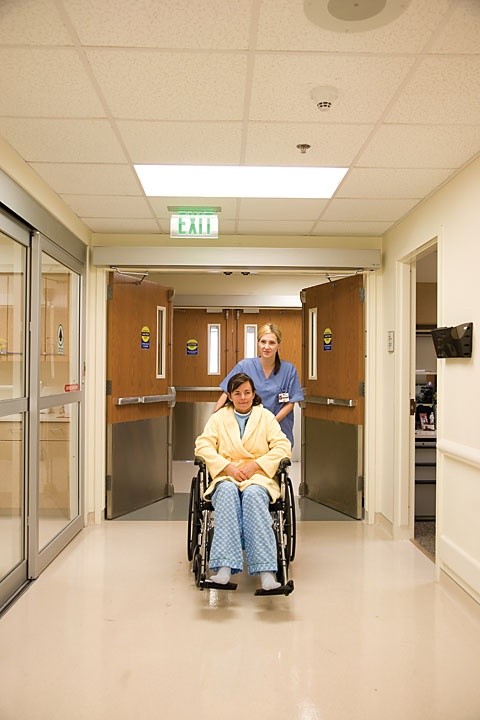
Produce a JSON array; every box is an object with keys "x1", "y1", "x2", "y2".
[
  {"x1": 0, "y1": 421, "x2": 23, "y2": 515},
  {"x1": 0, "y1": 272, "x2": 70, "y2": 364},
  {"x1": 39, "y1": 422, "x2": 71, "y2": 520},
  {"x1": 415, "y1": 425, "x2": 437, "y2": 522}
]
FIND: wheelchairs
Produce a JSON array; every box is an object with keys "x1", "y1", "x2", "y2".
[{"x1": 188, "y1": 457, "x2": 297, "y2": 595}]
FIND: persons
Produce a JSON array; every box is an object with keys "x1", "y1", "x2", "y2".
[
  {"x1": 213, "y1": 323, "x2": 304, "y2": 451},
  {"x1": 194, "y1": 372, "x2": 291, "y2": 590}
]
